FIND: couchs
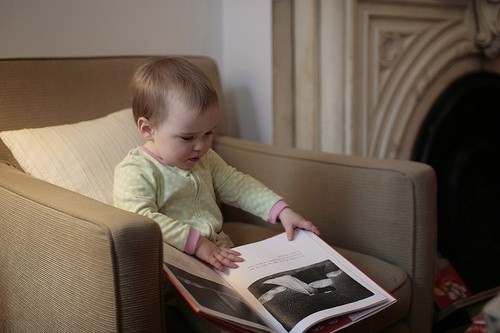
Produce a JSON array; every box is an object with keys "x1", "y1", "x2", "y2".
[{"x1": 1, "y1": 56, "x2": 438, "y2": 333}]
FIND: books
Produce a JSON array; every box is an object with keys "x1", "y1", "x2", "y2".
[{"x1": 162, "y1": 227, "x2": 397, "y2": 333}]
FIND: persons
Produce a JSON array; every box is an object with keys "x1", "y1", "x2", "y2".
[{"x1": 113, "y1": 55, "x2": 321, "y2": 272}]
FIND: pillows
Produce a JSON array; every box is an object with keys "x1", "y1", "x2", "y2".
[{"x1": 0, "y1": 107, "x2": 147, "y2": 207}]
